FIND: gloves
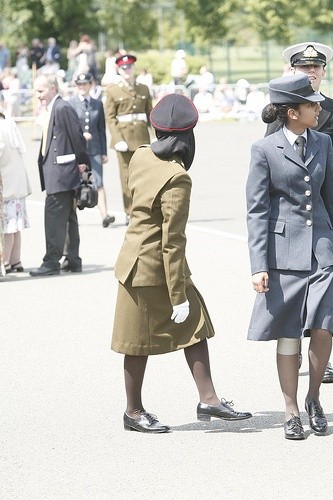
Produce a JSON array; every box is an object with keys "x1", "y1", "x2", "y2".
[{"x1": 114, "y1": 141, "x2": 128, "y2": 152}]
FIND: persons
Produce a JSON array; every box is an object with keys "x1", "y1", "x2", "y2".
[
  {"x1": 29, "y1": 73, "x2": 94, "y2": 277},
  {"x1": 264, "y1": 43, "x2": 332, "y2": 383},
  {"x1": 0, "y1": 34, "x2": 273, "y2": 228},
  {"x1": 110, "y1": 95, "x2": 252, "y2": 433},
  {"x1": 245, "y1": 73, "x2": 333, "y2": 440},
  {"x1": 0, "y1": 103, "x2": 32, "y2": 274}
]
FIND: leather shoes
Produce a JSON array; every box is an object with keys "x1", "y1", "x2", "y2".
[
  {"x1": 284, "y1": 412, "x2": 304, "y2": 439},
  {"x1": 321, "y1": 365, "x2": 333, "y2": 383},
  {"x1": 123, "y1": 411, "x2": 170, "y2": 434},
  {"x1": 304, "y1": 399, "x2": 328, "y2": 435},
  {"x1": 196, "y1": 398, "x2": 252, "y2": 422}
]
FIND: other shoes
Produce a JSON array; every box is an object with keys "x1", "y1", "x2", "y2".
[
  {"x1": 298, "y1": 340, "x2": 303, "y2": 369},
  {"x1": 103, "y1": 214, "x2": 115, "y2": 227},
  {"x1": 29, "y1": 262, "x2": 61, "y2": 276},
  {"x1": 61, "y1": 257, "x2": 82, "y2": 272}
]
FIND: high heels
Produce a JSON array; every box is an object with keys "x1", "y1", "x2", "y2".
[{"x1": 5, "y1": 261, "x2": 24, "y2": 273}]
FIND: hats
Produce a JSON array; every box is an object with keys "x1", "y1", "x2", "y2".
[
  {"x1": 268, "y1": 74, "x2": 325, "y2": 104},
  {"x1": 149, "y1": 93, "x2": 199, "y2": 171},
  {"x1": 115, "y1": 54, "x2": 138, "y2": 66},
  {"x1": 74, "y1": 74, "x2": 91, "y2": 84},
  {"x1": 282, "y1": 42, "x2": 333, "y2": 66}
]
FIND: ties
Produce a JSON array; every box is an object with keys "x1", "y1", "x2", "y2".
[{"x1": 296, "y1": 136, "x2": 306, "y2": 164}]
